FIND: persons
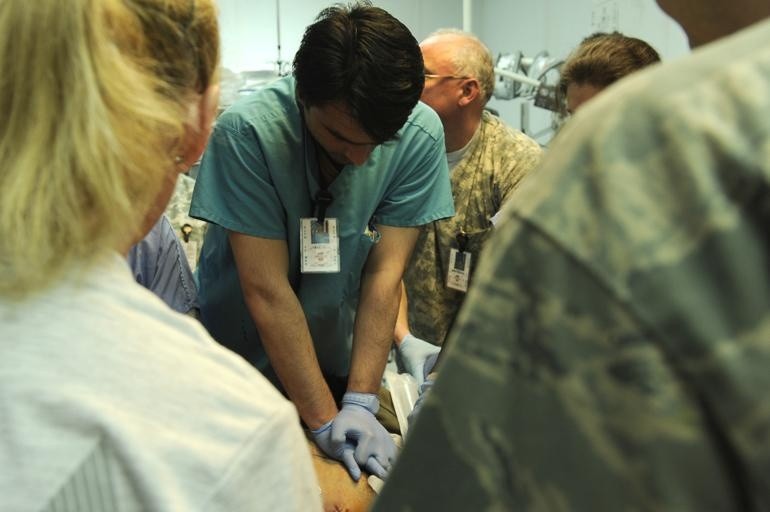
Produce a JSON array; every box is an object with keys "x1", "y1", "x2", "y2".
[
  {"x1": 390, "y1": 25, "x2": 546, "y2": 390},
  {"x1": 188, "y1": 2, "x2": 454, "y2": 482},
  {"x1": 0, "y1": 1, "x2": 323, "y2": 512},
  {"x1": 305, "y1": 434, "x2": 382, "y2": 511},
  {"x1": 365, "y1": 1, "x2": 770, "y2": 512},
  {"x1": 125, "y1": 211, "x2": 199, "y2": 319},
  {"x1": 556, "y1": 28, "x2": 661, "y2": 118}
]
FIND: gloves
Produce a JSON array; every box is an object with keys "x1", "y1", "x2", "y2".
[
  {"x1": 330, "y1": 392, "x2": 405, "y2": 472},
  {"x1": 313, "y1": 419, "x2": 391, "y2": 483},
  {"x1": 398, "y1": 335, "x2": 443, "y2": 392}
]
